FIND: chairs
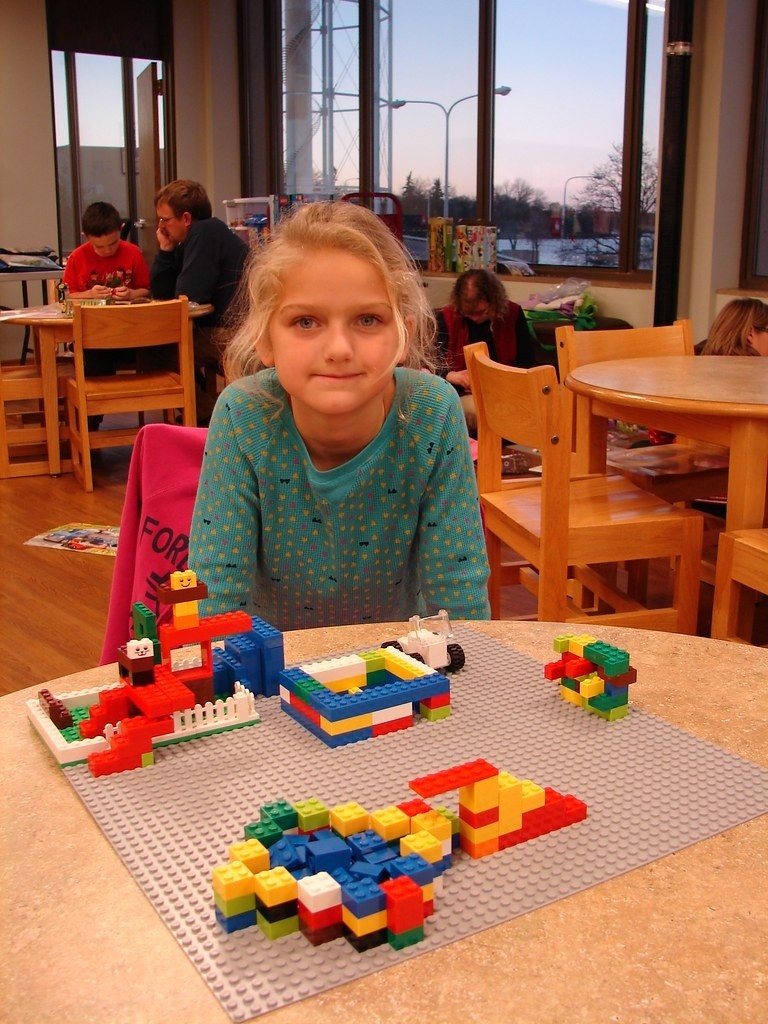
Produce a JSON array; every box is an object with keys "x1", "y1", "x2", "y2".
[
  {"x1": 66, "y1": 294, "x2": 197, "y2": 493},
  {"x1": 711, "y1": 528, "x2": 768, "y2": 644},
  {"x1": 1, "y1": 365, "x2": 76, "y2": 477},
  {"x1": 555, "y1": 319, "x2": 730, "y2": 608},
  {"x1": 463, "y1": 340, "x2": 705, "y2": 637}
]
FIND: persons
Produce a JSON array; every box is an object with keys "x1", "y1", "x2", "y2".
[
  {"x1": 675, "y1": 297, "x2": 768, "y2": 469},
  {"x1": 63, "y1": 200, "x2": 149, "y2": 455},
  {"x1": 186, "y1": 201, "x2": 492, "y2": 640},
  {"x1": 433, "y1": 268, "x2": 534, "y2": 448},
  {"x1": 149, "y1": 177, "x2": 252, "y2": 426}
]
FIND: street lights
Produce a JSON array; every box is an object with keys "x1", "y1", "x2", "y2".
[
  {"x1": 390, "y1": 85, "x2": 512, "y2": 218},
  {"x1": 561, "y1": 175, "x2": 605, "y2": 252}
]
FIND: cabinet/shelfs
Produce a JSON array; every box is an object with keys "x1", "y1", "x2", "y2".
[{"x1": 223, "y1": 194, "x2": 276, "y2": 255}]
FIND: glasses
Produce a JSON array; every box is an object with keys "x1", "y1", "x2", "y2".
[{"x1": 159, "y1": 215, "x2": 174, "y2": 222}]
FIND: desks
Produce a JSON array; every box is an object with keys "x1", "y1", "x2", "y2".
[
  {"x1": 564, "y1": 355, "x2": 768, "y2": 643},
  {"x1": 0, "y1": 620, "x2": 768, "y2": 1024},
  {"x1": 0, "y1": 302, "x2": 214, "y2": 477},
  {"x1": 0, "y1": 269, "x2": 65, "y2": 365}
]
